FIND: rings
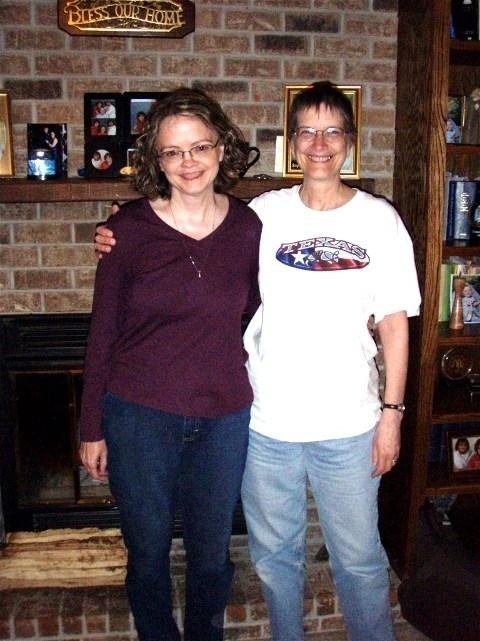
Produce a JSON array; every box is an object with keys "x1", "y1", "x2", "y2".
[{"x1": 392, "y1": 460, "x2": 398, "y2": 465}]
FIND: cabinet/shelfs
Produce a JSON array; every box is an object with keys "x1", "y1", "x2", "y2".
[{"x1": 393, "y1": 0, "x2": 480, "y2": 572}]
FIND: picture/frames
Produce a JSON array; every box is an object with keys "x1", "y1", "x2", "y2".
[
  {"x1": 446, "y1": 430, "x2": 480, "y2": 480},
  {"x1": 284, "y1": 86, "x2": 361, "y2": 180}
]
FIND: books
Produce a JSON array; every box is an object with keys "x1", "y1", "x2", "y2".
[{"x1": 438, "y1": 261, "x2": 480, "y2": 323}]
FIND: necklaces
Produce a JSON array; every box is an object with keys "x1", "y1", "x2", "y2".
[{"x1": 164, "y1": 194, "x2": 221, "y2": 281}]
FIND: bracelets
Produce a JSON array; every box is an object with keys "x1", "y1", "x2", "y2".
[{"x1": 381, "y1": 402, "x2": 407, "y2": 414}]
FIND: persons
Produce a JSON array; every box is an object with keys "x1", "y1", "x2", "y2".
[
  {"x1": 460, "y1": 285, "x2": 480, "y2": 323},
  {"x1": 88, "y1": 96, "x2": 158, "y2": 180},
  {"x1": 465, "y1": 439, "x2": 480, "y2": 471},
  {"x1": 445, "y1": 118, "x2": 460, "y2": 143},
  {"x1": 91, "y1": 79, "x2": 426, "y2": 641},
  {"x1": 453, "y1": 438, "x2": 474, "y2": 473},
  {"x1": 45, "y1": 131, "x2": 59, "y2": 152},
  {"x1": 42, "y1": 127, "x2": 50, "y2": 150},
  {"x1": 451, "y1": 277, "x2": 480, "y2": 318},
  {"x1": 77, "y1": 86, "x2": 377, "y2": 641}
]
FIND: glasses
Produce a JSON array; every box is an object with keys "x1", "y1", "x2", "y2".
[
  {"x1": 295, "y1": 127, "x2": 348, "y2": 140},
  {"x1": 156, "y1": 137, "x2": 219, "y2": 161}
]
